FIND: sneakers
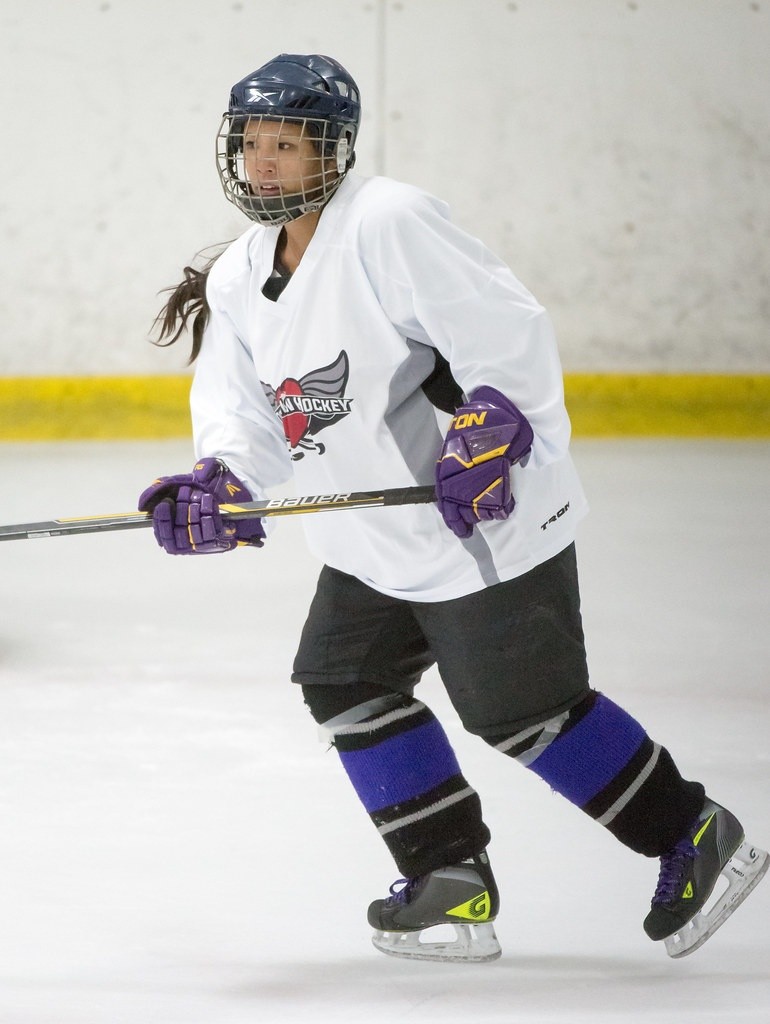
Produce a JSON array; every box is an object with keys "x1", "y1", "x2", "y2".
[
  {"x1": 643, "y1": 794, "x2": 770, "y2": 952},
  {"x1": 367, "y1": 848, "x2": 502, "y2": 962}
]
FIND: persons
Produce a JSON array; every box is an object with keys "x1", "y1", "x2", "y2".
[{"x1": 137, "y1": 54, "x2": 770, "y2": 964}]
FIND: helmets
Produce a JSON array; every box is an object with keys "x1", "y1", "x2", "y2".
[{"x1": 216, "y1": 52, "x2": 361, "y2": 229}]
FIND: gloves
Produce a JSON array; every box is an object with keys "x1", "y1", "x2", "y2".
[
  {"x1": 437, "y1": 383, "x2": 536, "y2": 537},
  {"x1": 139, "y1": 455, "x2": 267, "y2": 554}
]
